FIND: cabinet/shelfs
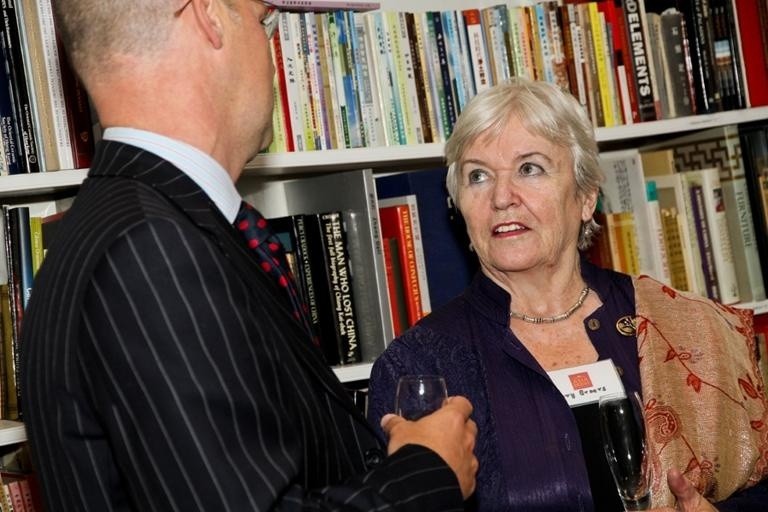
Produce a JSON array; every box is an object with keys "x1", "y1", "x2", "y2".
[{"x1": 0, "y1": 0, "x2": 768, "y2": 512}]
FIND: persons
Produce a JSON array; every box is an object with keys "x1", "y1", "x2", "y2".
[
  {"x1": 361, "y1": 74, "x2": 767, "y2": 511},
  {"x1": 19, "y1": 0, "x2": 482, "y2": 511}
]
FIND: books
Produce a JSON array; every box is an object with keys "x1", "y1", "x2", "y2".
[
  {"x1": 1, "y1": 444, "x2": 42, "y2": 512},
  {"x1": 256, "y1": 1, "x2": 765, "y2": 154},
  {"x1": 2, "y1": 2, "x2": 108, "y2": 176},
  {"x1": 2, "y1": 205, "x2": 70, "y2": 417},
  {"x1": 264, "y1": 117, "x2": 766, "y2": 367}
]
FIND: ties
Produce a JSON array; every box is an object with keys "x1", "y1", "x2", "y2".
[{"x1": 234, "y1": 201, "x2": 320, "y2": 349}]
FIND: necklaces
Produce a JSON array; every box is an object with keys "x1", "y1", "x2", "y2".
[{"x1": 508, "y1": 285, "x2": 589, "y2": 324}]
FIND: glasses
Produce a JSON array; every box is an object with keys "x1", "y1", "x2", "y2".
[{"x1": 173, "y1": 1, "x2": 280, "y2": 40}]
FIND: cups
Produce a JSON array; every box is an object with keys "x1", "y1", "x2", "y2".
[
  {"x1": 395, "y1": 373, "x2": 451, "y2": 425},
  {"x1": 597, "y1": 391, "x2": 652, "y2": 512}
]
